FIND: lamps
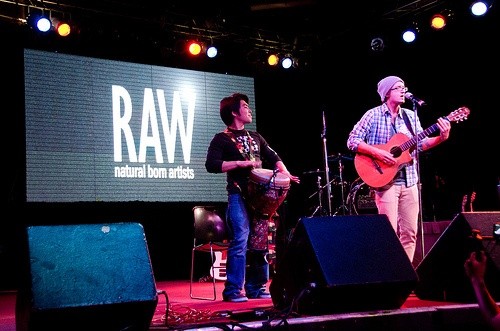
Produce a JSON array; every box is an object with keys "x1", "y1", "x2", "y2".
[
  {"x1": 431, "y1": 14, "x2": 446, "y2": 30},
  {"x1": 279, "y1": 56, "x2": 293, "y2": 69},
  {"x1": 471, "y1": 0, "x2": 487, "y2": 16},
  {"x1": 202, "y1": 45, "x2": 217, "y2": 58},
  {"x1": 51, "y1": 17, "x2": 70, "y2": 37},
  {"x1": 402, "y1": 22, "x2": 417, "y2": 43},
  {"x1": 30, "y1": 14, "x2": 52, "y2": 33},
  {"x1": 189, "y1": 43, "x2": 201, "y2": 55},
  {"x1": 265, "y1": 53, "x2": 279, "y2": 66}
]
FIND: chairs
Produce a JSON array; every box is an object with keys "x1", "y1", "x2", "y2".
[{"x1": 190, "y1": 206, "x2": 230, "y2": 301}]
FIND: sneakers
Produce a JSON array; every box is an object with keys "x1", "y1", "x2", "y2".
[
  {"x1": 258, "y1": 291, "x2": 271, "y2": 298},
  {"x1": 224, "y1": 293, "x2": 248, "y2": 302}
]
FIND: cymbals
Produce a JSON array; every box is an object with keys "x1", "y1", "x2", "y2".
[
  {"x1": 304, "y1": 171, "x2": 332, "y2": 175},
  {"x1": 328, "y1": 154, "x2": 354, "y2": 161}
]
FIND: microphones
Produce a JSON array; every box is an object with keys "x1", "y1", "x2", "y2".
[
  {"x1": 405, "y1": 91, "x2": 426, "y2": 106},
  {"x1": 273, "y1": 167, "x2": 281, "y2": 175}
]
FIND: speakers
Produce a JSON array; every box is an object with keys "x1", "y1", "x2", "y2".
[
  {"x1": 268, "y1": 214, "x2": 420, "y2": 313},
  {"x1": 411, "y1": 209, "x2": 499, "y2": 303},
  {"x1": 14, "y1": 223, "x2": 158, "y2": 331}
]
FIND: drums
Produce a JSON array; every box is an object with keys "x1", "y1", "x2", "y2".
[{"x1": 247, "y1": 169, "x2": 291, "y2": 219}]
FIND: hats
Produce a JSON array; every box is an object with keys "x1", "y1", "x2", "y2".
[{"x1": 376, "y1": 75, "x2": 404, "y2": 102}]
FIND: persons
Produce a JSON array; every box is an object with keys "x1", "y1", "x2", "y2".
[
  {"x1": 205, "y1": 93, "x2": 301, "y2": 303},
  {"x1": 347, "y1": 76, "x2": 451, "y2": 265},
  {"x1": 465, "y1": 251, "x2": 500, "y2": 329}
]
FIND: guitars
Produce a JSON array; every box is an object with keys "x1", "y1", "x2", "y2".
[
  {"x1": 354, "y1": 106, "x2": 470, "y2": 192},
  {"x1": 210, "y1": 240, "x2": 228, "y2": 280}
]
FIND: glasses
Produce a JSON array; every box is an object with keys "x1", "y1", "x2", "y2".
[{"x1": 389, "y1": 86, "x2": 408, "y2": 91}]
